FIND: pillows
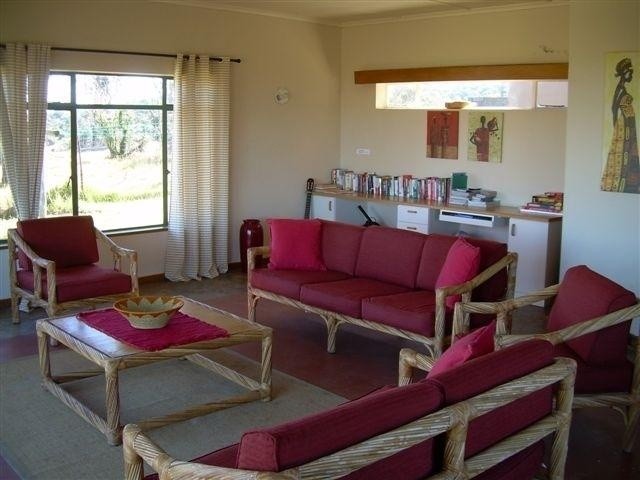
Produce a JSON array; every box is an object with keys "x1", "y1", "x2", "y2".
[
  {"x1": 435, "y1": 234, "x2": 480, "y2": 311},
  {"x1": 268, "y1": 219, "x2": 328, "y2": 272},
  {"x1": 421, "y1": 315, "x2": 497, "y2": 382}
]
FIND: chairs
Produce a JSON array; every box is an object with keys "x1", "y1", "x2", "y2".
[
  {"x1": 7, "y1": 213, "x2": 140, "y2": 348},
  {"x1": 448, "y1": 265, "x2": 639, "y2": 455}
]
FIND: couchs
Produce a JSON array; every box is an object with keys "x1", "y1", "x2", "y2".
[
  {"x1": 121, "y1": 339, "x2": 576, "y2": 480},
  {"x1": 246, "y1": 218, "x2": 520, "y2": 368}
]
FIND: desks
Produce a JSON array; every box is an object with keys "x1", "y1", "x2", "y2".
[{"x1": 310, "y1": 185, "x2": 563, "y2": 309}]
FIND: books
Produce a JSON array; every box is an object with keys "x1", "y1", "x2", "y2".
[{"x1": 312, "y1": 167, "x2": 563, "y2": 216}]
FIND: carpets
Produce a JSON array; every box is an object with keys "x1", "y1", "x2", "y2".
[{"x1": 1, "y1": 340, "x2": 352, "y2": 480}]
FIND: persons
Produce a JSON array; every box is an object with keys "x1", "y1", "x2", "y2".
[
  {"x1": 468, "y1": 114, "x2": 500, "y2": 163},
  {"x1": 601, "y1": 57, "x2": 639, "y2": 195},
  {"x1": 440, "y1": 116, "x2": 451, "y2": 158},
  {"x1": 427, "y1": 115, "x2": 440, "y2": 158}
]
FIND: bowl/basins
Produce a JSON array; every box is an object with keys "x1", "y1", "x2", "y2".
[
  {"x1": 113, "y1": 296, "x2": 185, "y2": 329},
  {"x1": 444, "y1": 101, "x2": 470, "y2": 109}
]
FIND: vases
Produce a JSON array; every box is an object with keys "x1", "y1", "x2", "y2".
[{"x1": 238, "y1": 219, "x2": 265, "y2": 275}]
